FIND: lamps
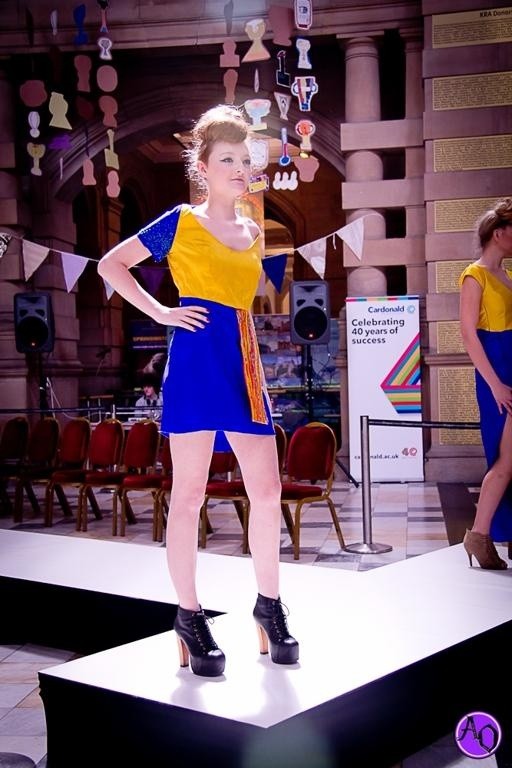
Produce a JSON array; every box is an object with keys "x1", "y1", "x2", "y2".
[
  {"x1": 280, "y1": 421, "x2": 345, "y2": 560},
  {"x1": 48, "y1": 418, "x2": 125, "y2": 527},
  {"x1": 1, "y1": 418, "x2": 60, "y2": 526},
  {"x1": 201, "y1": 423, "x2": 287, "y2": 554},
  {"x1": 157, "y1": 469, "x2": 172, "y2": 542},
  {"x1": 1, "y1": 417, "x2": 32, "y2": 476},
  {"x1": 18, "y1": 417, "x2": 91, "y2": 527},
  {"x1": 82, "y1": 420, "x2": 160, "y2": 536},
  {"x1": 119, "y1": 437, "x2": 172, "y2": 540}
]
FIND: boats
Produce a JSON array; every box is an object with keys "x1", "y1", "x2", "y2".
[
  {"x1": 13, "y1": 291, "x2": 57, "y2": 354},
  {"x1": 289, "y1": 282, "x2": 332, "y2": 347}
]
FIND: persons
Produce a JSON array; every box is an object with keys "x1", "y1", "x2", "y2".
[
  {"x1": 455, "y1": 198, "x2": 512, "y2": 571},
  {"x1": 95, "y1": 105, "x2": 300, "y2": 677},
  {"x1": 133, "y1": 376, "x2": 164, "y2": 421}
]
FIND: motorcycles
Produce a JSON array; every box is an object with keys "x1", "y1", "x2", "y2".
[{"x1": 281, "y1": 347, "x2": 361, "y2": 489}]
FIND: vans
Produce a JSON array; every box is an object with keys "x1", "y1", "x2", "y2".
[
  {"x1": 463, "y1": 528, "x2": 507, "y2": 570},
  {"x1": 253, "y1": 593, "x2": 299, "y2": 664},
  {"x1": 174, "y1": 604, "x2": 225, "y2": 677}
]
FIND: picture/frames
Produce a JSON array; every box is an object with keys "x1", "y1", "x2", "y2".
[{"x1": 86, "y1": 418, "x2": 161, "y2": 474}]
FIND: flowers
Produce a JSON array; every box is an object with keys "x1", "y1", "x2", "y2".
[{"x1": 95, "y1": 347, "x2": 110, "y2": 357}]
